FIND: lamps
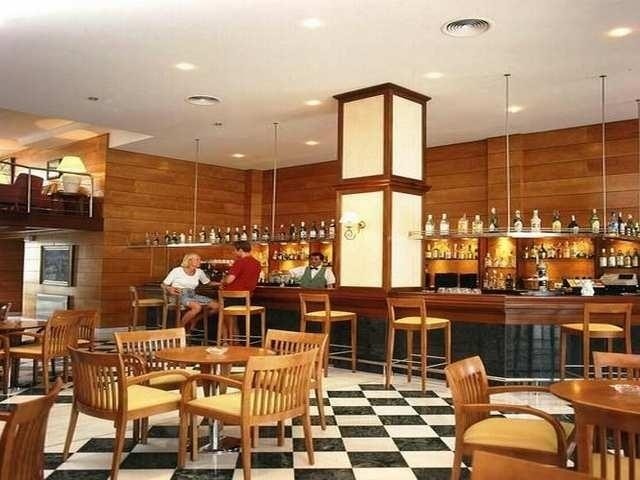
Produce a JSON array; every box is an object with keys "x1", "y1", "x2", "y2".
[
  {"x1": 339, "y1": 210, "x2": 367, "y2": 240},
  {"x1": 56, "y1": 156, "x2": 88, "y2": 193}
]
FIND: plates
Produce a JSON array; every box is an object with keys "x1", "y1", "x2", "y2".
[
  {"x1": 205, "y1": 346, "x2": 229, "y2": 357},
  {"x1": 610, "y1": 384, "x2": 640, "y2": 395}
]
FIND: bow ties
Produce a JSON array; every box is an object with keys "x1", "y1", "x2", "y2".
[{"x1": 310, "y1": 267, "x2": 318, "y2": 270}]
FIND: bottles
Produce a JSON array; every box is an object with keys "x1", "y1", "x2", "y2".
[
  {"x1": 198, "y1": 250, "x2": 308, "y2": 287},
  {"x1": 423, "y1": 206, "x2": 640, "y2": 297},
  {"x1": 143, "y1": 218, "x2": 338, "y2": 246}
]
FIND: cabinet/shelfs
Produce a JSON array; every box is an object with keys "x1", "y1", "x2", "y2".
[
  {"x1": 413, "y1": 215, "x2": 636, "y2": 287},
  {"x1": 151, "y1": 239, "x2": 336, "y2": 280}
]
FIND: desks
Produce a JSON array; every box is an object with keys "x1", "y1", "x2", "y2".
[
  {"x1": 546, "y1": 378, "x2": 640, "y2": 431},
  {"x1": 1, "y1": 316, "x2": 47, "y2": 388},
  {"x1": 155, "y1": 344, "x2": 277, "y2": 460}
]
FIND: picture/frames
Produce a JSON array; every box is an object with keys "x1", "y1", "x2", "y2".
[
  {"x1": 39, "y1": 245, "x2": 74, "y2": 287},
  {"x1": 46, "y1": 159, "x2": 62, "y2": 180}
]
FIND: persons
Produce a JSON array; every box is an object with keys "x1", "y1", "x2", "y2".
[
  {"x1": 267, "y1": 251, "x2": 336, "y2": 329},
  {"x1": 222, "y1": 240, "x2": 261, "y2": 346},
  {"x1": 160, "y1": 252, "x2": 223, "y2": 333}
]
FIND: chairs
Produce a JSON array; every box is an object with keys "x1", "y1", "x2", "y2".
[
  {"x1": 41, "y1": 183, "x2": 57, "y2": 197},
  {"x1": 592, "y1": 349, "x2": 640, "y2": 382},
  {"x1": 443, "y1": 354, "x2": 575, "y2": 480},
  {"x1": 248, "y1": 328, "x2": 330, "y2": 444},
  {"x1": 36, "y1": 307, "x2": 99, "y2": 365},
  {"x1": 113, "y1": 328, "x2": 205, "y2": 446},
  {"x1": 59, "y1": 343, "x2": 201, "y2": 480},
  {"x1": 2, "y1": 379, "x2": 63, "y2": 479},
  {"x1": 573, "y1": 398, "x2": 639, "y2": 479},
  {"x1": 177, "y1": 347, "x2": 318, "y2": 480},
  {"x1": 469, "y1": 448, "x2": 598, "y2": 478},
  {"x1": 0, "y1": 309, "x2": 85, "y2": 398},
  {"x1": 0, "y1": 173, "x2": 43, "y2": 212}
]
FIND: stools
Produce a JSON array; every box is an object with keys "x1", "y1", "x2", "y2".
[
  {"x1": 295, "y1": 290, "x2": 359, "y2": 379},
  {"x1": 211, "y1": 290, "x2": 268, "y2": 350},
  {"x1": 384, "y1": 295, "x2": 454, "y2": 394},
  {"x1": 558, "y1": 300, "x2": 635, "y2": 380},
  {"x1": 125, "y1": 286, "x2": 162, "y2": 333},
  {"x1": 160, "y1": 286, "x2": 210, "y2": 345}
]
